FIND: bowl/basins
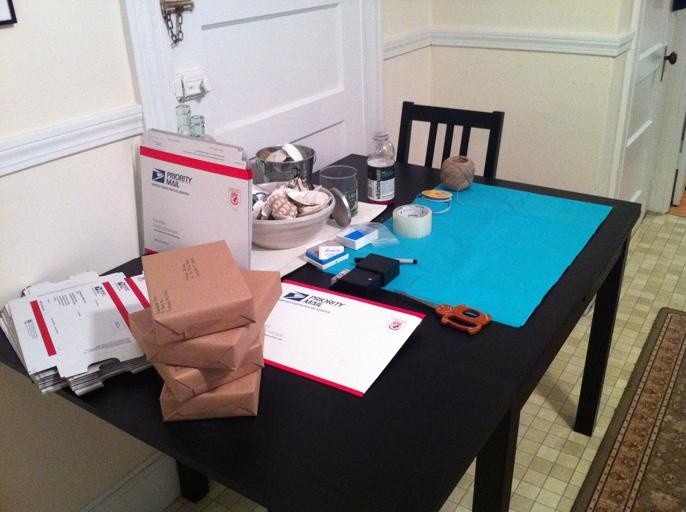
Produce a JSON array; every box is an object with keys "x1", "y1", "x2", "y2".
[{"x1": 249, "y1": 180, "x2": 335, "y2": 251}]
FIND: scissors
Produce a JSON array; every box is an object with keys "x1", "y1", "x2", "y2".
[{"x1": 395, "y1": 290, "x2": 491, "y2": 335}]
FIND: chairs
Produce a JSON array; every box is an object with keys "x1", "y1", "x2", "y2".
[{"x1": 398, "y1": 101, "x2": 505, "y2": 178}]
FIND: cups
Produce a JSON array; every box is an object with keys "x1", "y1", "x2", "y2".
[
  {"x1": 252, "y1": 145, "x2": 316, "y2": 183},
  {"x1": 317, "y1": 164, "x2": 360, "y2": 220}
]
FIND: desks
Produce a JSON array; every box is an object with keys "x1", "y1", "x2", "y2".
[{"x1": 0, "y1": 153, "x2": 642, "y2": 512}]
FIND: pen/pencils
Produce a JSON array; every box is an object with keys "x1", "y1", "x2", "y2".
[{"x1": 354, "y1": 258, "x2": 417, "y2": 263}]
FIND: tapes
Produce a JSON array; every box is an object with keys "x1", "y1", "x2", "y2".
[{"x1": 392, "y1": 204, "x2": 432, "y2": 239}]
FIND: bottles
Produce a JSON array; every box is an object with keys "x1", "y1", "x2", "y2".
[
  {"x1": 367, "y1": 128, "x2": 398, "y2": 209},
  {"x1": 173, "y1": 104, "x2": 206, "y2": 141}
]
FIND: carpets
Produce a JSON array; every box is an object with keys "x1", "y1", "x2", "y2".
[{"x1": 571, "y1": 307, "x2": 686, "y2": 511}]
